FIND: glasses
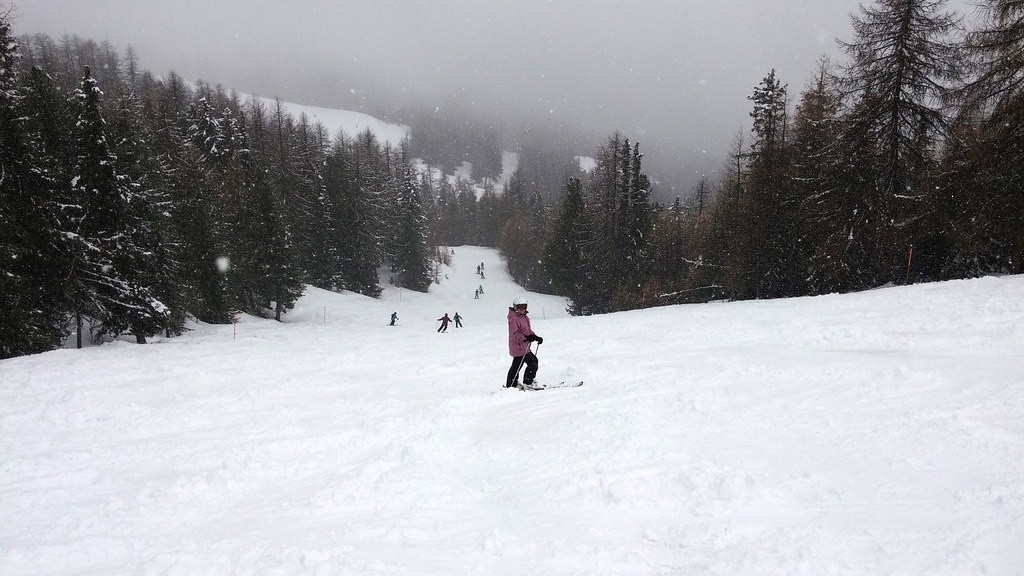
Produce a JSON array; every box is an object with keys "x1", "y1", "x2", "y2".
[{"x1": 517, "y1": 304, "x2": 527, "y2": 309}]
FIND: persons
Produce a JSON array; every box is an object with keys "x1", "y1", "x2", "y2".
[
  {"x1": 390, "y1": 312, "x2": 399, "y2": 325},
  {"x1": 453, "y1": 312, "x2": 463, "y2": 328},
  {"x1": 437, "y1": 313, "x2": 453, "y2": 333},
  {"x1": 474, "y1": 285, "x2": 484, "y2": 299},
  {"x1": 506, "y1": 296, "x2": 545, "y2": 390},
  {"x1": 476, "y1": 262, "x2": 485, "y2": 279}
]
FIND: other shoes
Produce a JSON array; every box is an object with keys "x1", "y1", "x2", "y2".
[
  {"x1": 523, "y1": 380, "x2": 541, "y2": 389},
  {"x1": 516, "y1": 384, "x2": 523, "y2": 390}
]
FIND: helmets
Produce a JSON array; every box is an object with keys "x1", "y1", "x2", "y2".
[{"x1": 512, "y1": 297, "x2": 528, "y2": 308}]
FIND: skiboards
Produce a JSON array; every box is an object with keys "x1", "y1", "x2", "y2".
[
  {"x1": 387, "y1": 323, "x2": 400, "y2": 326},
  {"x1": 438, "y1": 331, "x2": 449, "y2": 333},
  {"x1": 502, "y1": 380, "x2": 584, "y2": 391}
]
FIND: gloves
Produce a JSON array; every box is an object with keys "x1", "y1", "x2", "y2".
[
  {"x1": 534, "y1": 335, "x2": 543, "y2": 344},
  {"x1": 526, "y1": 335, "x2": 535, "y2": 342}
]
